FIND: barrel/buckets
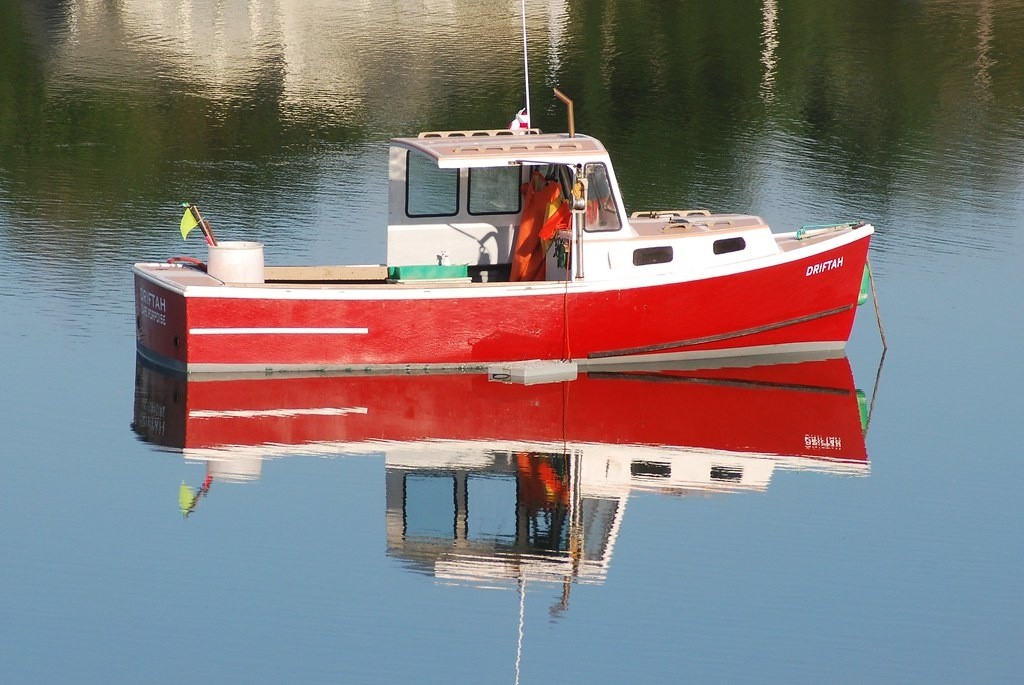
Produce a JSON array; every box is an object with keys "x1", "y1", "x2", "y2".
[
  {"x1": 207, "y1": 241, "x2": 264, "y2": 283},
  {"x1": 205, "y1": 456, "x2": 264, "y2": 484}
]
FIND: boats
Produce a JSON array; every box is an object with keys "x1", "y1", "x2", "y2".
[
  {"x1": 129, "y1": 351, "x2": 874, "y2": 610},
  {"x1": 131, "y1": 87, "x2": 875, "y2": 373}
]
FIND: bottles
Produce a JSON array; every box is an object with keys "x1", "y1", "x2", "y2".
[
  {"x1": 519, "y1": 111, "x2": 528, "y2": 129},
  {"x1": 440, "y1": 249, "x2": 449, "y2": 266}
]
